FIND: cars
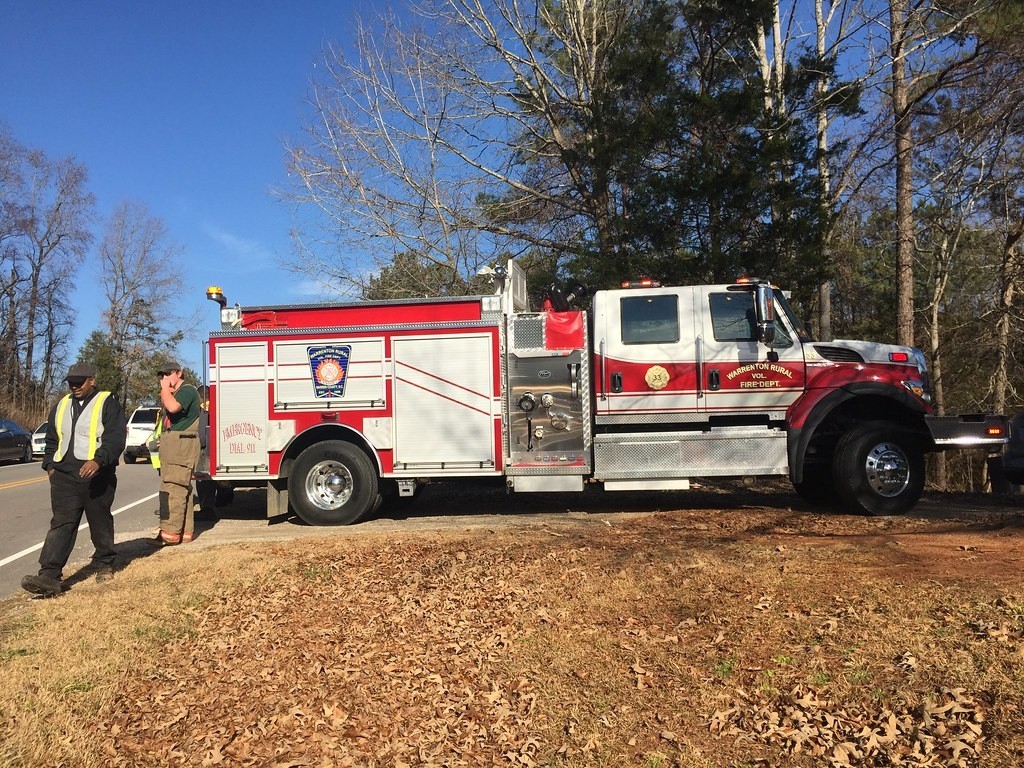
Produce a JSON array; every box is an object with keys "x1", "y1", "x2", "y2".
[
  {"x1": 0, "y1": 418, "x2": 32, "y2": 463},
  {"x1": 30, "y1": 421, "x2": 48, "y2": 454}
]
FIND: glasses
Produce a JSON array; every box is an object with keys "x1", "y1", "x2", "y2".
[{"x1": 161, "y1": 372, "x2": 171, "y2": 377}]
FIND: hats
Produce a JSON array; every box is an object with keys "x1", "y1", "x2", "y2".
[
  {"x1": 157, "y1": 362, "x2": 181, "y2": 376},
  {"x1": 62, "y1": 362, "x2": 95, "y2": 383}
]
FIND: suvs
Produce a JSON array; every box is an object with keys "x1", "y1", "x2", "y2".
[{"x1": 123, "y1": 406, "x2": 163, "y2": 464}]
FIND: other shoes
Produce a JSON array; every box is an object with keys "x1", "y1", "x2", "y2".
[
  {"x1": 96, "y1": 567, "x2": 114, "y2": 584},
  {"x1": 21, "y1": 573, "x2": 61, "y2": 598},
  {"x1": 146, "y1": 535, "x2": 179, "y2": 546}
]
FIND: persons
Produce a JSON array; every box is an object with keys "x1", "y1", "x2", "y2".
[
  {"x1": 21, "y1": 362, "x2": 126, "y2": 596},
  {"x1": 146, "y1": 361, "x2": 237, "y2": 546}
]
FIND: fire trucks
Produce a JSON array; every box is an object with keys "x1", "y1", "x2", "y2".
[{"x1": 200, "y1": 260, "x2": 1013, "y2": 527}]
